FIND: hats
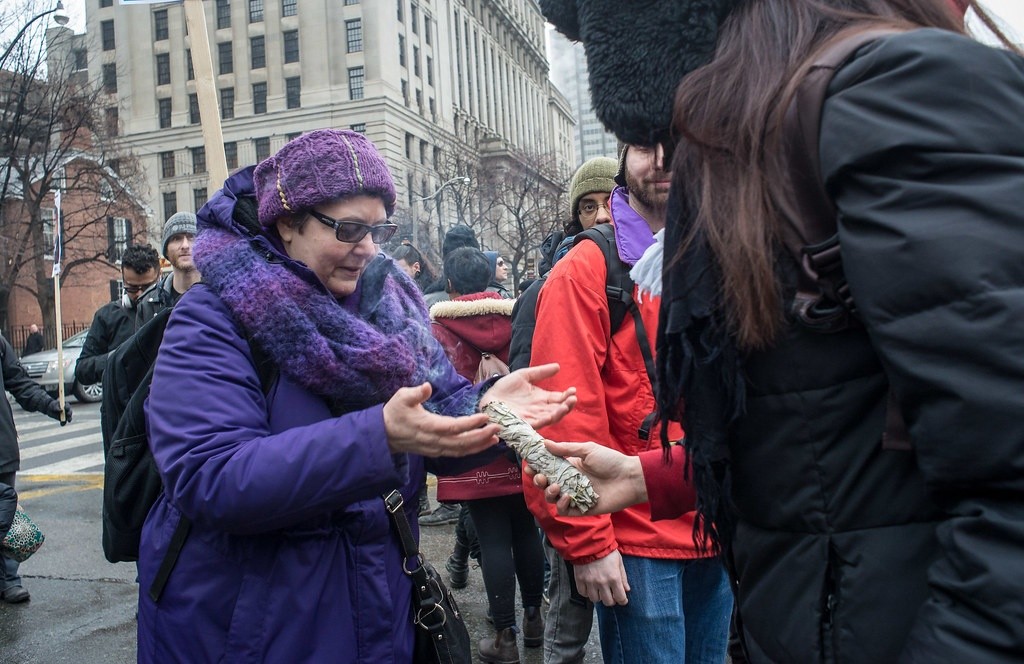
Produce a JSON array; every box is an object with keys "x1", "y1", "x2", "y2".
[
  {"x1": 443, "y1": 226, "x2": 479, "y2": 261},
  {"x1": 163, "y1": 211, "x2": 200, "y2": 261},
  {"x1": 543, "y1": 0, "x2": 727, "y2": 145},
  {"x1": 252, "y1": 126, "x2": 399, "y2": 238},
  {"x1": 568, "y1": 157, "x2": 621, "y2": 219}
]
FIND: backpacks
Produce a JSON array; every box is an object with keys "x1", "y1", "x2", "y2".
[
  {"x1": 99, "y1": 282, "x2": 278, "y2": 563},
  {"x1": 472, "y1": 353, "x2": 510, "y2": 387}
]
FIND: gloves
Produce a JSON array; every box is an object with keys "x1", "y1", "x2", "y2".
[{"x1": 46, "y1": 401, "x2": 74, "y2": 423}]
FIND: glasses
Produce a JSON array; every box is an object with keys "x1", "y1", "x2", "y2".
[
  {"x1": 497, "y1": 260, "x2": 504, "y2": 267},
  {"x1": 301, "y1": 205, "x2": 397, "y2": 244},
  {"x1": 123, "y1": 278, "x2": 160, "y2": 294},
  {"x1": 577, "y1": 202, "x2": 610, "y2": 217}
]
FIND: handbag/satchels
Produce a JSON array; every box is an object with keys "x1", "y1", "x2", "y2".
[
  {"x1": 412, "y1": 565, "x2": 472, "y2": 663},
  {"x1": 2, "y1": 507, "x2": 45, "y2": 562}
]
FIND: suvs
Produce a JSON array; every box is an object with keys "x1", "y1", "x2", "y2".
[{"x1": 19, "y1": 327, "x2": 104, "y2": 412}]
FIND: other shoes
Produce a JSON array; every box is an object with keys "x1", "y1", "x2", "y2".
[
  {"x1": 1, "y1": 586, "x2": 30, "y2": 604},
  {"x1": 543, "y1": 583, "x2": 552, "y2": 605}
]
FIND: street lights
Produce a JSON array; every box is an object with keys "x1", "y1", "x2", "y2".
[{"x1": 410, "y1": 177, "x2": 471, "y2": 249}]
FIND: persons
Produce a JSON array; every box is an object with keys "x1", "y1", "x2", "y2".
[
  {"x1": 22, "y1": 324, "x2": 44, "y2": 357},
  {"x1": 73, "y1": 131, "x2": 735, "y2": 664},
  {"x1": 539, "y1": 0, "x2": 1024, "y2": 664},
  {"x1": 0, "y1": 328, "x2": 73, "y2": 603},
  {"x1": 53, "y1": 205, "x2": 59, "y2": 264}
]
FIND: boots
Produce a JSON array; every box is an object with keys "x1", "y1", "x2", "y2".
[
  {"x1": 446, "y1": 539, "x2": 469, "y2": 588},
  {"x1": 417, "y1": 501, "x2": 462, "y2": 525},
  {"x1": 524, "y1": 607, "x2": 545, "y2": 647},
  {"x1": 415, "y1": 484, "x2": 432, "y2": 516},
  {"x1": 477, "y1": 626, "x2": 520, "y2": 664},
  {"x1": 485, "y1": 606, "x2": 496, "y2": 624}
]
